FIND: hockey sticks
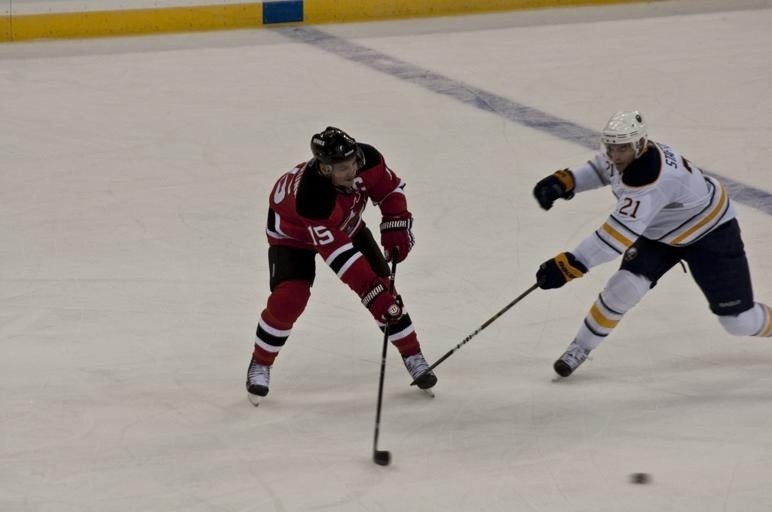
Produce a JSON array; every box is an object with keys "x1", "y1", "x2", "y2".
[
  {"x1": 374, "y1": 246, "x2": 400, "y2": 465},
  {"x1": 410, "y1": 282, "x2": 540, "y2": 385}
]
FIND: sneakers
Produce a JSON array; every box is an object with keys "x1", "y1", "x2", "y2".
[
  {"x1": 247, "y1": 352, "x2": 270, "y2": 395},
  {"x1": 403, "y1": 349, "x2": 437, "y2": 389},
  {"x1": 554, "y1": 338, "x2": 591, "y2": 376}
]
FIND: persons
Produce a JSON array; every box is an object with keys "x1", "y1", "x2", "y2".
[
  {"x1": 533, "y1": 107, "x2": 771, "y2": 378},
  {"x1": 245, "y1": 126, "x2": 437, "y2": 397}
]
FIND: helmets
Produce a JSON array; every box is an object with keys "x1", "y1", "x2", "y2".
[
  {"x1": 602, "y1": 109, "x2": 649, "y2": 159},
  {"x1": 311, "y1": 125, "x2": 366, "y2": 178}
]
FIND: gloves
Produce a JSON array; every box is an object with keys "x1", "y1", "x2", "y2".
[
  {"x1": 534, "y1": 168, "x2": 576, "y2": 210},
  {"x1": 380, "y1": 214, "x2": 416, "y2": 263},
  {"x1": 536, "y1": 250, "x2": 588, "y2": 289},
  {"x1": 359, "y1": 276, "x2": 403, "y2": 325}
]
